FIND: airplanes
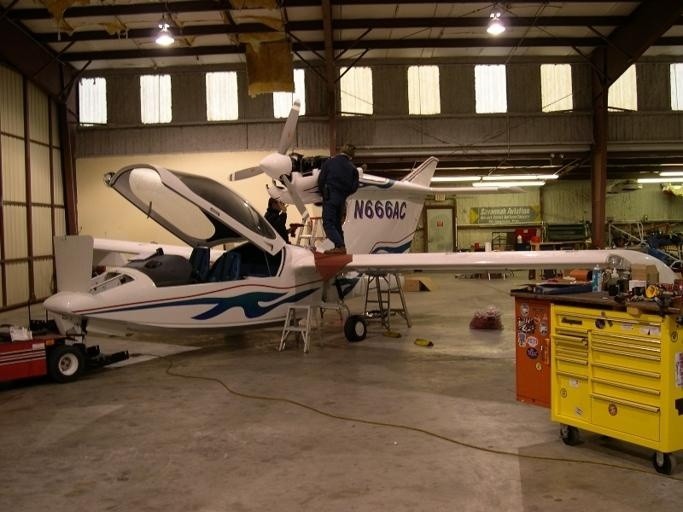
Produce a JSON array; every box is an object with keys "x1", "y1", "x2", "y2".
[{"x1": 38, "y1": 97, "x2": 674, "y2": 383}]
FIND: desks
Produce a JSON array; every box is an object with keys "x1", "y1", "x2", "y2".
[
  {"x1": 508, "y1": 291, "x2": 550, "y2": 408},
  {"x1": 547, "y1": 295, "x2": 682, "y2": 475}
]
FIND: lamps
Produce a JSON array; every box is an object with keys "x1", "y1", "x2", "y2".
[
  {"x1": 153, "y1": 11, "x2": 175, "y2": 48},
  {"x1": 484, "y1": 1, "x2": 506, "y2": 37}
]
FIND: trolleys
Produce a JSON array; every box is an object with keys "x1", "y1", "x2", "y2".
[{"x1": 506, "y1": 269, "x2": 682, "y2": 474}]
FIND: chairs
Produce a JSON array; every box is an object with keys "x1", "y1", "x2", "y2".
[
  {"x1": 211, "y1": 250, "x2": 242, "y2": 283},
  {"x1": 187, "y1": 246, "x2": 211, "y2": 287}
]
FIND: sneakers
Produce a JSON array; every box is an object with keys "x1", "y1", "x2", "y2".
[{"x1": 325, "y1": 247, "x2": 346, "y2": 254}]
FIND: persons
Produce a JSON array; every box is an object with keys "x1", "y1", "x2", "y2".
[
  {"x1": 264, "y1": 196, "x2": 291, "y2": 245},
  {"x1": 317, "y1": 143, "x2": 360, "y2": 255}
]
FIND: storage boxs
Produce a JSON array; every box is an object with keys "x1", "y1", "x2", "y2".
[{"x1": 402, "y1": 276, "x2": 434, "y2": 291}]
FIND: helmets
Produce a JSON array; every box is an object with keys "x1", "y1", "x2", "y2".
[{"x1": 340, "y1": 144, "x2": 355, "y2": 158}]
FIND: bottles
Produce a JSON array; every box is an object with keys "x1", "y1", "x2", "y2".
[{"x1": 591, "y1": 255, "x2": 682, "y2": 302}]
